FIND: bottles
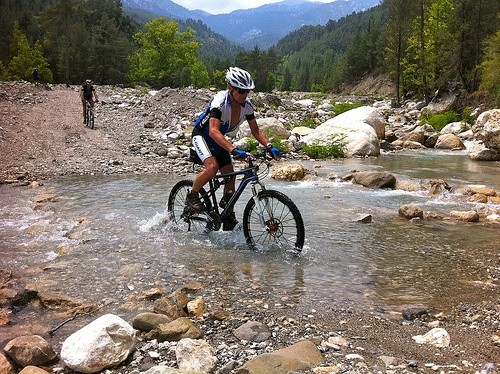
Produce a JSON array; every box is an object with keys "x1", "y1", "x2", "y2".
[{"x1": 219, "y1": 190, "x2": 233, "y2": 209}]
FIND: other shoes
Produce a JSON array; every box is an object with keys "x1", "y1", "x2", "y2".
[
  {"x1": 185, "y1": 191, "x2": 206, "y2": 211},
  {"x1": 218, "y1": 197, "x2": 228, "y2": 208}
]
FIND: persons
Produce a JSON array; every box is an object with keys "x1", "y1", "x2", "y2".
[
  {"x1": 80, "y1": 79, "x2": 98, "y2": 124},
  {"x1": 185, "y1": 65, "x2": 281, "y2": 215}
]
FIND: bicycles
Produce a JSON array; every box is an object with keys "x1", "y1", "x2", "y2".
[
  {"x1": 167, "y1": 146, "x2": 306, "y2": 258},
  {"x1": 84, "y1": 100, "x2": 99, "y2": 129}
]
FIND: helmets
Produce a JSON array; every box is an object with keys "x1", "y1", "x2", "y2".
[{"x1": 224, "y1": 66, "x2": 256, "y2": 89}]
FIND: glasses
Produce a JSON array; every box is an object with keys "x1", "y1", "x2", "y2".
[{"x1": 233, "y1": 87, "x2": 250, "y2": 94}]
